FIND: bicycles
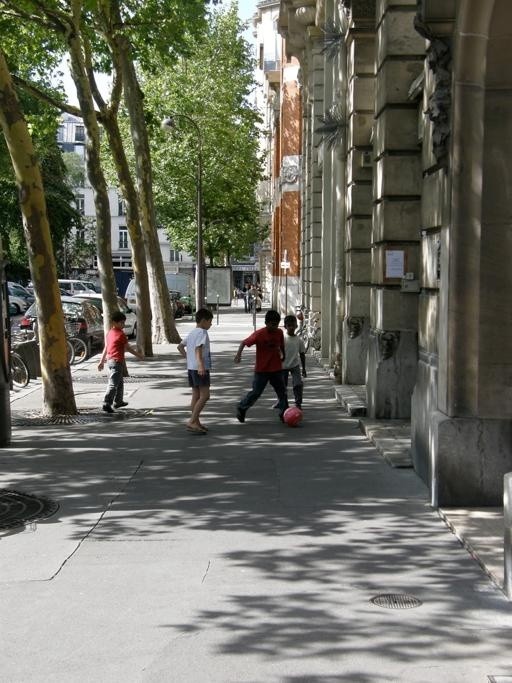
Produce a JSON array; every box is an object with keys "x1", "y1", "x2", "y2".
[
  {"x1": 0, "y1": 314, "x2": 89, "y2": 389},
  {"x1": 293, "y1": 304, "x2": 322, "y2": 353}
]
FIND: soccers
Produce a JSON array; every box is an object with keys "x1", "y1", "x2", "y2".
[{"x1": 283, "y1": 406, "x2": 303, "y2": 428}]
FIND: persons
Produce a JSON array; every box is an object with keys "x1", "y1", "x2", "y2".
[
  {"x1": 233, "y1": 281, "x2": 264, "y2": 313},
  {"x1": 176, "y1": 307, "x2": 214, "y2": 435},
  {"x1": 272, "y1": 313, "x2": 307, "y2": 409},
  {"x1": 234, "y1": 310, "x2": 289, "y2": 423},
  {"x1": 97, "y1": 312, "x2": 143, "y2": 412}
]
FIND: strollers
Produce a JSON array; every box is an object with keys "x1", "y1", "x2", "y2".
[{"x1": 246, "y1": 288, "x2": 262, "y2": 313}]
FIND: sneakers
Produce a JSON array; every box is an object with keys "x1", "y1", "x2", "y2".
[
  {"x1": 235, "y1": 414, "x2": 244, "y2": 422},
  {"x1": 196, "y1": 424, "x2": 209, "y2": 431},
  {"x1": 114, "y1": 402, "x2": 127, "y2": 408},
  {"x1": 295, "y1": 404, "x2": 303, "y2": 413},
  {"x1": 273, "y1": 402, "x2": 289, "y2": 422},
  {"x1": 187, "y1": 424, "x2": 206, "y2": 434},
  {"x1": 103, "y1": 405, "x2": 113, "y2": 412}
]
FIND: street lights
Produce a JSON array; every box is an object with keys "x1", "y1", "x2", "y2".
[{"x1": 161, "y1": 113, "x2": 204, "y2": 322}]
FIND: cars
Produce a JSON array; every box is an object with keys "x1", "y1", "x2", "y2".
[{"x1": 0, "y1": 277, "x2": 196, "y2": 359}]
